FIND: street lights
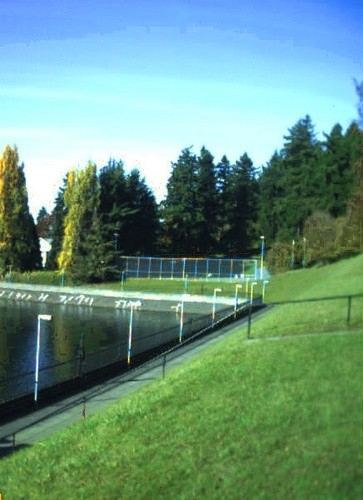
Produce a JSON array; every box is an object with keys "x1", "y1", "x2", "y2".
[
  {"x1": 180, "y1": 294, "x2": 191, "y2": 344},
  {"x1": 128, "y1": 300, "x2": 143, "y2": 371},
  {"x1": 251, "y1": 282, "x2": 257, "y2": 306},
  {"x1": 235, "y1": 284, "x2": 244, "y2": 321},
  {"x1": 35, "y1": 314, "x2": 52, "y2": 407},
  {"x1": 213, "y1": 289, "x2": 222, "y2": 330},
  {"x1": 261, "y1": 236, "x2": 265, "y2": 281},
  {"x1": 263, "y1": 281, "x2": 271, "y2": 304}
]
